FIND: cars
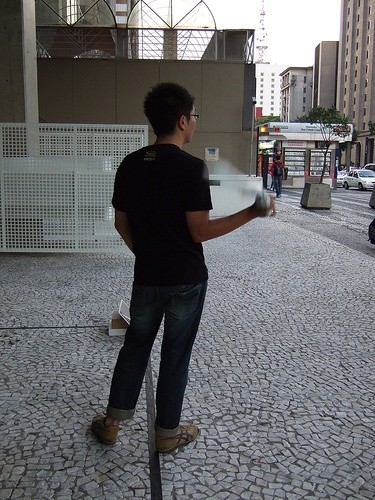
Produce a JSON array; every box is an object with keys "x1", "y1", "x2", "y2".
[
  {"x1": 362, "y1": 164, "x2": 375, "y2": 173},
  {"x1": 343, "y1": 169, "x2": 375, "y2": 190},
  {"x1": 337, "y1": 170, "x2": 343, "y2": 187}
]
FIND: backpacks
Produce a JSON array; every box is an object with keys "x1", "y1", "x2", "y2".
[{"x1": 274, "y1": 163, "x2": 283, "y2": 177}]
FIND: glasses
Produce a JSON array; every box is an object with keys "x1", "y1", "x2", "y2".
[{"x1": 185, "y1": 114, "x2": 199, "y2": 120}]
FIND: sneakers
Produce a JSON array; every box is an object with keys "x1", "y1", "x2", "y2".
[
  {"x1": 92, "y1": 414, "x2": 120, "y2": 445},
  {"x1": 154, "y1": 425, "x2": 199, "y2": 453}
]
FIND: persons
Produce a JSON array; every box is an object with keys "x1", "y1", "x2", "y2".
[
  {"x1": 270, "y1": 154, "x2": 286, "y2": 198},
  {"x1": 90, "y1": 81, "x2": 277, "y2": 454}
]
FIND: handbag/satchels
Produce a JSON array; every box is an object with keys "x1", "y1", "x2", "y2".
[{"x1": 368, "y1": 217, "x2": 375, "y2": 244}]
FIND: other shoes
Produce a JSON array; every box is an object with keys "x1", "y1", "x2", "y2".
[{"x1": 277, "y1": 193, "x2": 281, "y2": 198}]
